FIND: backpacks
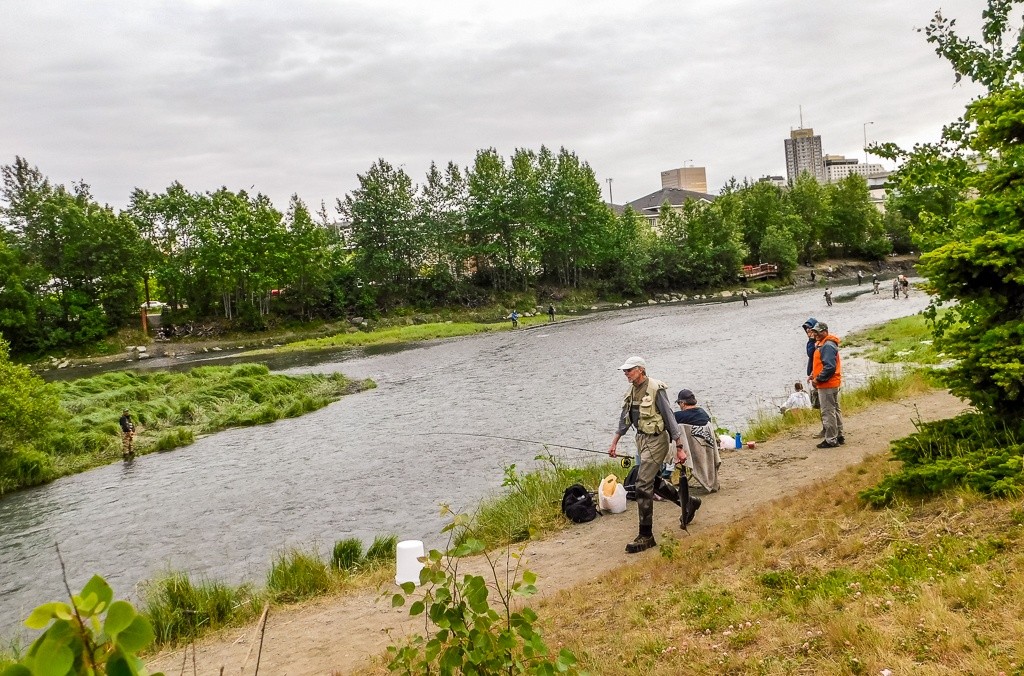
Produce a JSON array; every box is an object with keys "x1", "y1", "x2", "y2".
[
  {"x1": 562, "y1": 484, "x2": 603, "y2": 523},
  {"x1": 623, "y1": 465, "x2": 639, "y2": 501}
]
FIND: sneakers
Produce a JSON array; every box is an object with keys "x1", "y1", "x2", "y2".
[
  {"x1": 625, "y1": 534, "x2": 657, "y2": 553},
  {"x1": 679, "y1": 496, "x2": 701, "y2": 524}
]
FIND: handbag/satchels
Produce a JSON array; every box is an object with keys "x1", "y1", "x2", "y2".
[
  {"x1": 598, "y1": 474, "x2": 628, "y2": 514},
  {"x1": 809, "y1": 392, "x2": 820, "y2": 409}
]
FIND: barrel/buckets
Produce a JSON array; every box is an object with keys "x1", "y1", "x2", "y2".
[
  {"x1": 736, "y1": 433, "x2": 742, "y2": 449},
  {"x1": 395, "y1": 540, "x2": 425, "y2": 586},
  {"x1": 718, "y1": 435, "x2": 736, "y2": 448}
]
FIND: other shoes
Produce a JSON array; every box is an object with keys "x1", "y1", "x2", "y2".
[
  {"x1": 816, "y1": 439, "x2": 839, "y2": 448},
  {"x1": 812, "y1": 429, "x2": 826, "y2": 438},
  {"x1": 837, "y1": 435, "x2": 845, "y2": 445}
]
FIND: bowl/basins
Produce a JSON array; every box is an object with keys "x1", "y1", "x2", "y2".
[{"x1": 747, "y1": 442, "x2": 755, "y2": 449}]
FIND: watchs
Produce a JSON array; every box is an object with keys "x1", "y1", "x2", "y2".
[{"x1": 676, "y1": 444, "x2": 683, "y2": 448}]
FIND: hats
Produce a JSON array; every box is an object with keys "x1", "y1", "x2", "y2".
[
  {"x1": 675, "y1": 389, "x2": 694, "y2": 403},
  {"x1": 617, "y1": 356, "x2": 645, "y2": 370},
  {"x1": 123, "y1": 409, "x2": 129, "y2": 413},
  {"x1": 812, "y1": 322, "x2": 828, "y2": 332}
]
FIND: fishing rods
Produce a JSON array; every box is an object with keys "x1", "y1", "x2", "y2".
[
  {"x1": 743, "y1": 389, "x2": 781, "y2": 408},
  {"x1": 418, "y1": 431, "x2": 635, "y2": 469}
]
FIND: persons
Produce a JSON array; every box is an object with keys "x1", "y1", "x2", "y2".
[
  {"x1": 607, "y1": 356, "x2": 702, "y2": 554},
  {"x1": 824, "y1": 287, "x2": 832, "y2": 306},
  {"x1": 548, "y1": 303, "x2": 555, "y2": 322},
  {"x1": 872, "y1": 274, "x2": 880, "y2": 294},
  {"x1": 742, "y1": 289, "x2": 749, "y2": 306},
  {"x1": 801, "y1": 316, "x2": 819, "y2": 408},
  {"x1": 779, "y1": 382, "x2": 812, "y2": 415},
  {"x1": 512, "y1": 309, "x2": 518, "y2": 327},
  {"x1": 901, "y1": 277, "x2": 910, "y2": 298},
  {"x1": 807, "y1": 320, "x2": 846, "y2": 449},
  {"x1": 858, "y1": 271, "x2": 864, "y2": 286},
  {"x1": 662, "y1": 388, "x2": 710, "y2": 479},
  {"x1": 119, "y1": 408, "x2": 136, "y2": 459},
  {"x1": 892, "y1": 279, "x2": 899, "y2": 299},
  {"x1": 828, "y1": 265, "x2": 833, "y2": 277},
  {"x1": 811, "y1": 270, "x2": 816, "y2": 281}
]
